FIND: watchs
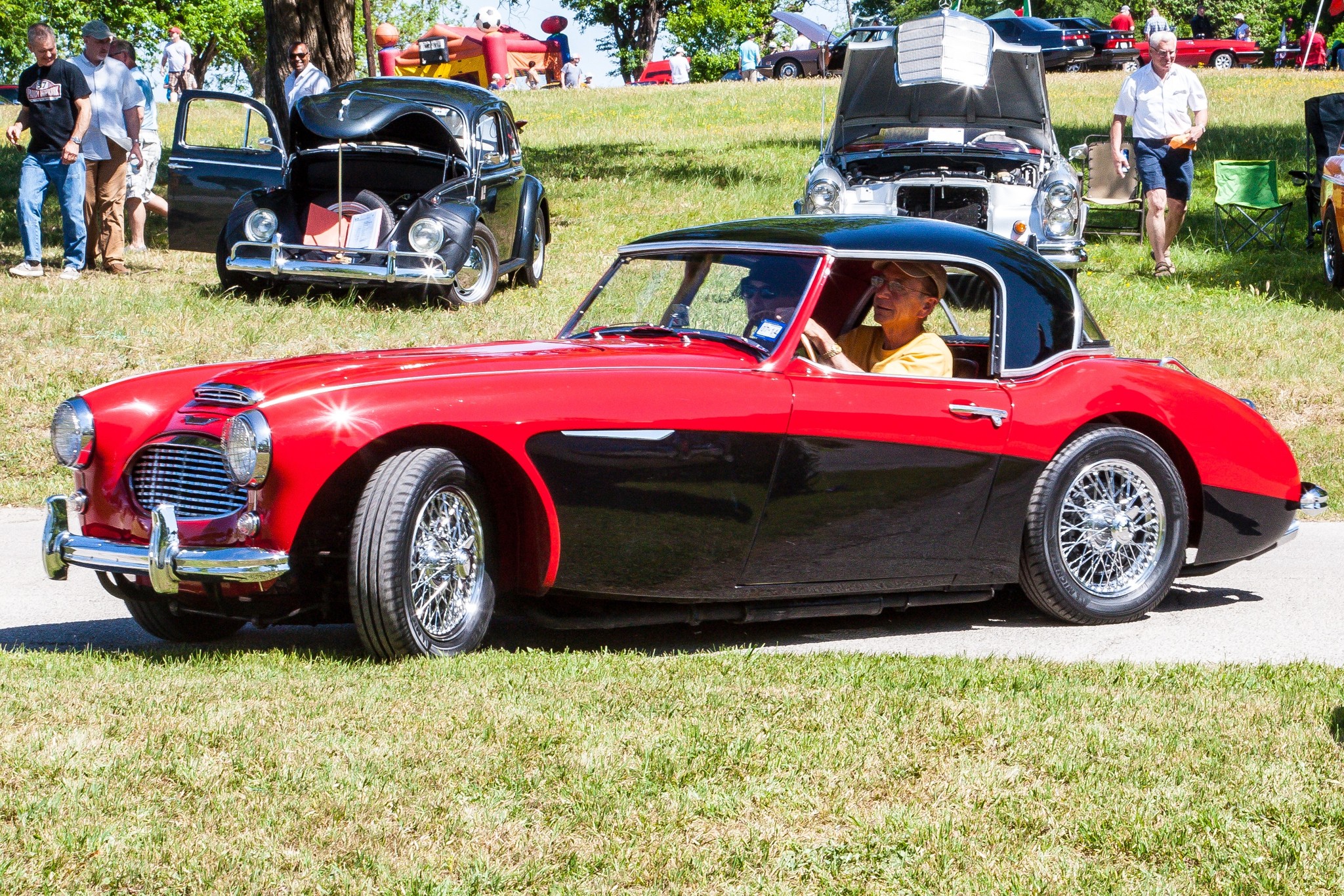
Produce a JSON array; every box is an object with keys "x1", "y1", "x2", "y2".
[
  {"x1": 1196, "y1": 124, "x2": 1206, "y2": 133},
  {"x1": 70, "y1": 136, "x2": 81, "y2": 144},
  {"x1": 821, "y1": 342, "x2": 842, "y2": 359}
]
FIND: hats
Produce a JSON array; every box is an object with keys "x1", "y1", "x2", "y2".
[
  {"x1": 780, "y1": 42, "x2": 790, "y2": 47},
  {"x1": 585, "y1": 74, "x2": 593, "y2": 79},
  {"x1": 871, "y1": 260, "x2": 948, "y2": 302},
  {"x1": 492, "y1": 73, "x2": 502, "y2": 80},
  {"x1": 768, "y1": 42, "x2": 777, "y2": 48},
  {"x1": 169, "y1": 27, "x2": 180, "y2": 34},
  {"x1": 739, "y1": 257, "x2": 810, "y2": 298},
  {"x1": 571, "y1": 53, "x2": 581, "y2": 58},
  {"x1": 676, "y1": 46, "x2": 687, "y2": 54},
  {"x1": 505, "y1": 74, "x2": 512, "y2": 79},
  {"x1": 1121, "y1": 5, "x2": 1130, "y2": 12},
  {"x1": 747, "y1": 34, "x2": 755, "y2": 39},
  {"x1": 1305, "y1": 22, "x2": 1313, "y2": 29},
  {"x1": 1234, "y1": 13, "x2": 1245, "y2": 21},
  {"x1": 82, "y1": 20, "x2": 114, "y2": 40}
]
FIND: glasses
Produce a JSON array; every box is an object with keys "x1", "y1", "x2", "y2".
[
  {"x1": 1150, "y1": 46, "x2": 1178, "y2": 57},
  {"x1": 870, "y1": 275, "x2": 932, "y2": 298},
  {"x1": 109, "y1": 51, "x2": 124, "y2": 58},
  {"x1": 288, "y1": 52, "x2": 308, "y2": 60},
  {"x1": 739, "y1": 285, "x2": 778, "y2": 300}
]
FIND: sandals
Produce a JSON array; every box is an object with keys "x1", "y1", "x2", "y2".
[
  {"x1": 1150, "y1": 250, "x2": 1175, "y2": 273},
  {"x1": 1153, "y1": 263, "x2": 1172, "y2": 277}
]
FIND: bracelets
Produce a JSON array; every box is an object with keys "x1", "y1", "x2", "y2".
[
  {"x1": 132, "y1": 139, "x2": 141, "y2": 146},
  {"x1": 739, "y1": 68, "x2": 741, "y2": 70}
]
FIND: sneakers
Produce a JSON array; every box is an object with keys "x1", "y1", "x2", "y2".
[
  {"x1": 60, "y1": 267, "x2": 80, "y2": 281},
  {"x1": 10, "y1": 262, "x2": 44, "y2": 277},
  {"x1": 106, "y1": 263, "x2": 131, "y2": 275}
]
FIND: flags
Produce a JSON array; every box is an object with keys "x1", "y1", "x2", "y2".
[
  {"x1": 1014, "y1": 0, "x2": 1030, "y2": 17},
  {"x1": 1275, "y1": 22, "x2": 1287, "y2": 68}
]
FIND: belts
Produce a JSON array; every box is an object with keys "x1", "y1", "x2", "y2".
[{"x1": 1135, "y1": 136, "x2": 1172, "y2": 144}]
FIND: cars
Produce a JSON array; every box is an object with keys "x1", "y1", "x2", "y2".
[
  {"x1": 756, "y1": 9, "x2": 899, "y2": 81},
  {"x1": 1308, "y1": 125, "x2": 1344, "y2": 289},
  {"x1": 983, "y1": 16, "x2": 1267, "y2": 74},
  {"x1": 791, "y1": 9, "x2": 1090, "y2": 270},
  {"x1": 167, "y1": 73, "x2": 553, "y2": 309},
  {"x1": 41, "y1": 216, "x2": 1330, "y2": 660}
]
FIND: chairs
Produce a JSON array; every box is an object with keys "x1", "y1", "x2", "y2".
[
  {"x1": 1304, "y1": 92, "x2": 1344, "y2": 250},
  {"x1": 1080, "y1": 135, "x2": 1145, "y2": 245},
  {"x1": 1213, "y1": 159, "x2": 1292, "y2": 251}
]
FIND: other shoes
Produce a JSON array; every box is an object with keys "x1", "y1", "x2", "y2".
[{"x1": 125, "y1": 243, "x2": 147, "y2": 252}]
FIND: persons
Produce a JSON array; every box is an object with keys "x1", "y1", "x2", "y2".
[
  {"x1": 1144, "y1": 8, "x2": 1170, "y2": 42},
  {"x1": 159, "y1": 27, "x2": 198, "y2": 102},
  {"x1": 1234, "y1": 13, "x2": 1252, "y2": 68},
  {"x1": 1190, "y1": 5, "x2": 1214, "y2": 39},
  {"x1": 1110, "y1": 31, "x2": 1208, "y2": 276},
  {"x1": 107, "y1": 39, "x2": 168, "y2": 254},
  {"x1": 6, "y1": 23, "x2": 93, "y2": 280},
  {"x1": 284, "y1": 41, "x2": 331, "y2": 119},
  {"x1": 738, "y1": 256, "x2": 809, "y2": 326},
  {"x1": 487, "y1": 53, "x2": 595, "y2": 91},
  {"x1": 768, "y1": 17, "x2": 888, "y2": 54},
  {"x1": 1110, "y1": 5, "x2": 1135, "y2": 31},
  {"x1": 66, "y1": 20, "x2": 144, "y2": 275},
  {"x1": 1295, "y1": 22, "x2": 1327, "y2": 71},
  {"x1": 669, "y1": 46, "x2": 690, "y2": 85},
  {"x1": 738, "y1": 33, "x2": 761, "y2": 83},
  {"x1": 775, "y1": 259, "x2": 953, "y2": 378}
]
